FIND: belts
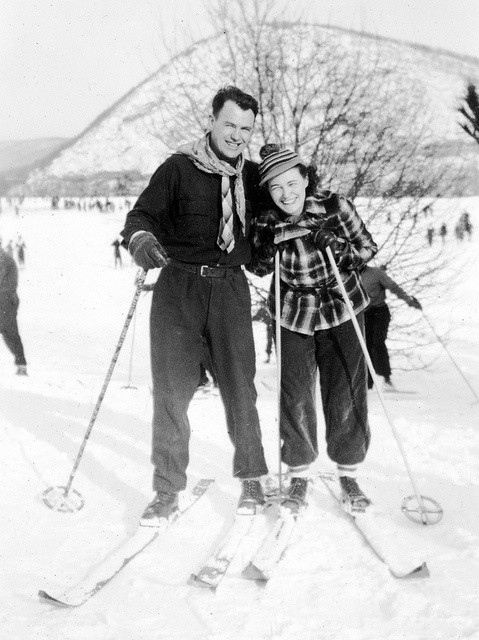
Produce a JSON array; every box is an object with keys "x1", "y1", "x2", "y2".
[{"x1": 170, "y1": 260, "x2": 240, "y2": 276}]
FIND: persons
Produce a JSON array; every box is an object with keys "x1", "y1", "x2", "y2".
[
  {"x1": 356, "y1": 266, "x2": 421, "y2": 385},
  {"x1": 247, "y1": 144, "x2": 376, "y2": 513},
  {"x1": 14, "y1": 235, "x2": 26, "y2": 266},
  {"x1": 119, "y1": 85, "x2": 274, "y2": 528},
  {"x1": 0, "y1": 243, "x2": 29, "y2": 375},
  {"x1": 426, "y1": 210, "x2": 473, "y2": 246},
  {"x1": 111, "y1": 238, "x2": 123, "y2": 266}
]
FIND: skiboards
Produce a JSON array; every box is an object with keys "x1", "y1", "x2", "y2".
[
  {"x1": 241, "y1": 473, "x2": 428, "y2": 586},
  {"x1": 38, "y1": 478, "x2": 272, "y2": 607}
]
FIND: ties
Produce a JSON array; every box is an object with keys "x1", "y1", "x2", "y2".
[{"x1": 217, "y1": 174, "x2": 237, "y2": 256}]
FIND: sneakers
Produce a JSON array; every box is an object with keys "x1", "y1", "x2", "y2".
[
  {"x1": 236, "y1": 480, "x2": 264, "y2": 514},
  {"x1": 288, "y1": 478, "x2": 306, "y2": 497},
  {"x1": 140, "y1": 492, "x2": 179, "y2": 526},
  {"x1": 340, "y1": 477, "x2": 359, "y2": 496}
]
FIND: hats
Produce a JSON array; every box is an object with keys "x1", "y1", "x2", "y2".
[{"x1": 258, "y1": 144, "x2": 301, "y2": 187}]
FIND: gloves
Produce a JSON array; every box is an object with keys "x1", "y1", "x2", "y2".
[
  {"x1": 256, "y1": 239, "x2": 277, "y2": 261},
  {"x1": 129, "y1": 232, "x2": 168, "y2": 269},
  {"x1": 314, "y1": 228, "x2": 336, "y2": 251},
  {"x1": 409, "y1": 296, "x2": 422, "y2": 309}
]
